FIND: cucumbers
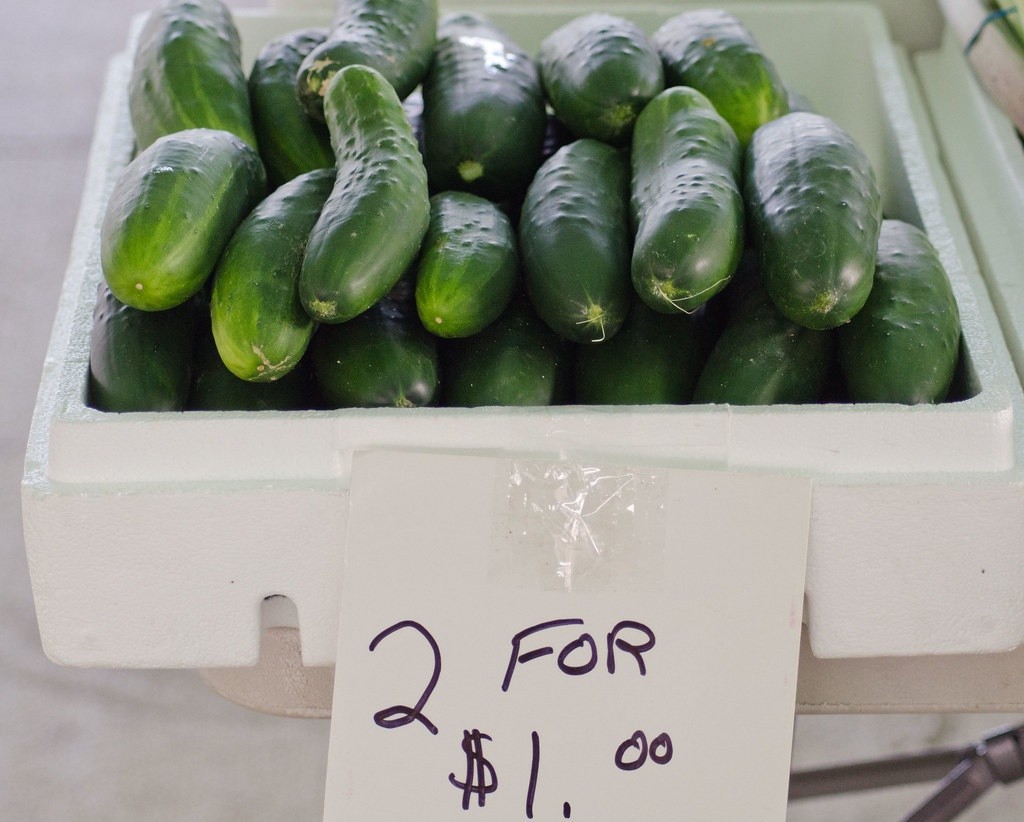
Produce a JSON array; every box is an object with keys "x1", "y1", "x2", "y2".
[{"x1": 88, "y1": 1, "x2": 964, "y2": 406}]
